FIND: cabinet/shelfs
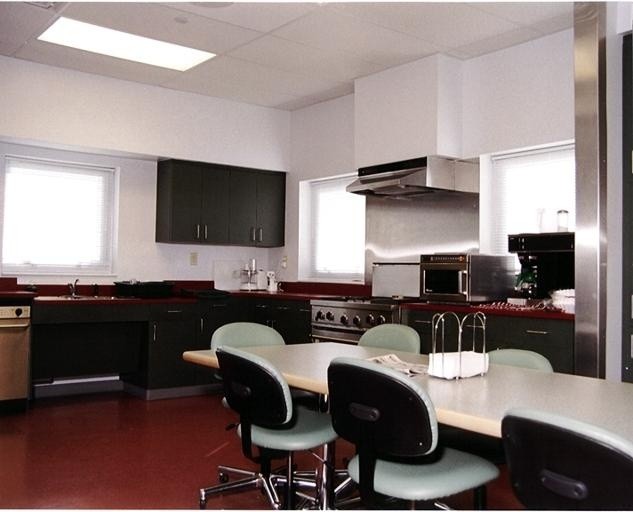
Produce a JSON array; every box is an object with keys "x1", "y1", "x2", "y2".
[
  {"x1": 229, "y1": 166, "x2": 286, "y2": 248},
  {"x1": 120, "y1": 298, "x2": 311, "y2": 401},
  {"x1": 154, "y1": 158, "x2": 229, "y2": 247},
  {"x1": 405, "y1": 308, "x2": 574, "y2": 375}
]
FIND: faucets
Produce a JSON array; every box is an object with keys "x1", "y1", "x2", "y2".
[{"x1": 67, "y1": 278, "x2": 80, "y2": 299}]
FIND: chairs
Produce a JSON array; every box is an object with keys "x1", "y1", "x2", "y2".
[
  {"x1": 358, "y1": 324, "x2": 420, "y2": 353},
  {"x1": 501, "y1": 406, "x2": 633, "y2": 511},
  {"x1": 211, "y1": 322, "x2": 285, "y2": 351},
  {"x1": 198, "y1": 344, "x2": 340, "y2": 510},
  {"x1": 328, "y1": 357, "x2": 500, "y2": 511},
  {"x1": 487, "y1": 348, "x2": 553, "y2": 374}
]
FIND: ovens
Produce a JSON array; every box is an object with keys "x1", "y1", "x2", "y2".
[{"x1": 309, "y1": 327, "x2": 363, "y2": 345}]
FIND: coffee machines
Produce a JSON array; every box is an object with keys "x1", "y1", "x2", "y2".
[{"x1": 506, "y1": 231, "x2": 573, "y2": 300}]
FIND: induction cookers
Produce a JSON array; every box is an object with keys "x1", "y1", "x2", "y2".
[{"x1": 310, "y1": 296, "x2": 416, "y2": 334}]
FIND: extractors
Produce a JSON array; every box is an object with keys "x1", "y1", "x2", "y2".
[{"x1": 346, "y1": 156, "x2": 479, "y2": 201}]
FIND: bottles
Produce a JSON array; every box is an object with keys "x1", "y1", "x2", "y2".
[
  {"x1": 556, "y1": 209, "x2": 568, "y2": 232},
  {"x1": 240, "y1": 258, "x2": 257, "y2": 290}
]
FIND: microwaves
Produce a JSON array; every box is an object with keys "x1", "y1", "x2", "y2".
[{"x1": 421, "y1": 252, "x2": 516, "y2": 306}]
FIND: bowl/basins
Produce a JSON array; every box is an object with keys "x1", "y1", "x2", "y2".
[{"x1": 111, "y1": 280, "x2": 174, "y2": 297}]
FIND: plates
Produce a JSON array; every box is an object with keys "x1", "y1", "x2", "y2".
[{"x1": 552, "y1": 289, "x2": 575, "y2": 315}]
